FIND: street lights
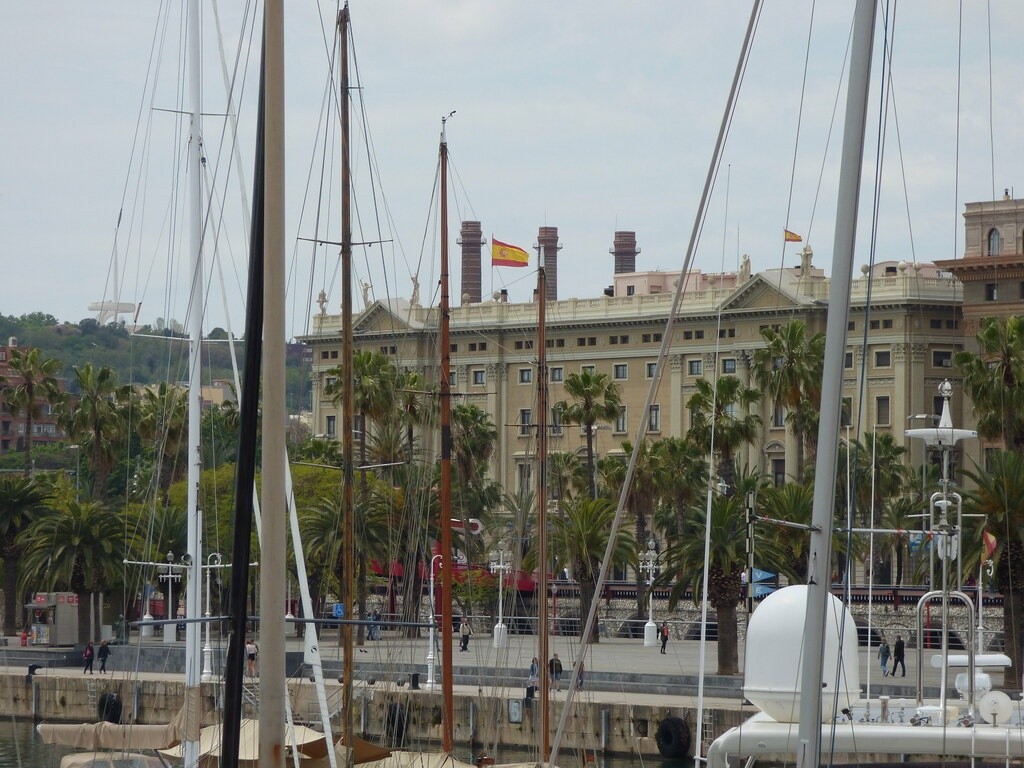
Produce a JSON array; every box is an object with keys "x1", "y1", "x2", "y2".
[
  {"x1": 70, "y1": 444, "x2": 79, "y2": 501},
  {"x1": 551, "y1": 584, "x2": 558, "y2": 635},
  {"x1": 488, "y1": 539, "x2": 513, "y2": 648},
  {"x1": 201, "y1": 553, "x2": 222, "y2": 682},
  {"x1": 638, "y1": 539, "x2": 658, "y2": 647},
  {"x1": 977, "y1": 559, "x2": 994, "y2": 655},
  {"x1": 426, "y1": 555, "x2": 442, "y2": 691},
  {"x1": 156, "y1": 551, "x2": 183, "y2": 620}
]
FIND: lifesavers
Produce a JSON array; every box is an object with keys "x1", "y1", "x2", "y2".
[
  {"x1": 657, "y1": 718, "x2": 690, "y2": 759},
  {"x1": 99, "y1": 694, "x2": 122, "y2": 723},
  {"x1": 384, "y1": 703, "x2": 408, "y2": 737}
]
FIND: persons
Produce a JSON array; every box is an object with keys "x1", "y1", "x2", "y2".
[
  {"x1": 97, "y1": 640, "x2": 111, "y2": 674},
  {"x1": 529, "y1": 657, "x2": 538, "y2": 692},
  {"x1": 549, "y1": 653, "x2": 562, "y2": 692},
  {"x1": 84, "y1": 640, "x2": 94, "y2": 675},
  {"x1": 890, "y1": 634, "x2": 905, "y2": 677},
  {"x1": 878, "y1": 637, "x2": 892, "y2": 676},
  {"x1": 366, "y1": 610, "x2": 382, "y2": 641},
  {"x1": 456, "y1": 618, "x2": 472, "y2": 652},
  {"x1": 433, "y1": 619, "x2": 441, "y2": 652},
  {"x1": 245, "y1": 639, "x2": 259, "y2": 677},
  {"x1": 660, "y1": 624, "x2": 669, "y2": 654},
  {"x1": 573, "y1": 655, "x2": 584, "y2": 692}
]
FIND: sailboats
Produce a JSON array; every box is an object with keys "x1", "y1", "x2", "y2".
[{"x1": 0, "y1": 0, "x2": 1024, "y2": 768}]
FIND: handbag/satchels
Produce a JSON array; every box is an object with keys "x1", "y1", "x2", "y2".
[
  {"x1": 84, "y1": 647, "x2": 90, "y2": 656},
  {"x1": 529, "y1": 675, "x2": 536, "y2": 682}
]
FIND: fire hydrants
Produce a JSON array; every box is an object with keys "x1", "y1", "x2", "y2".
[{"x1": 20, "y1": 632, "x2": 27, "y2": 646}]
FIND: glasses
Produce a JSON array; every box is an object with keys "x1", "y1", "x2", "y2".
[{"x1": 534, "y1": 660, "x2": 537, "y2": 661}]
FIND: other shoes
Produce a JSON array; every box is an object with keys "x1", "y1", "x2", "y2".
[
  {"x1": 550, "y1": 687, "x2": 552, "y2": 691},
  {"x1": 885, "y1": 671, "x2": 889, "y2": 676},
  {"x1": 663, "y1": 652, "x2": 666, "y2": 654},
  {"x1": 901, "y1": 675, "x2": 905, "y2": 677},
  {"x1": 437, "y1": 650, "x2": 442, "y2": 652},
  {"x1": 460, "y1": 646, "x2": 463, "y2": 652},
  {"x1": 466, "y1": 649, "x2": 470, "y2": 652},
  {"x1": 880, "y1": 672, "x2": 885, "y2": 675},
  {"x1": 890, "y1": 673, "x2": 894, "y2": 676},
  {"x1": 661, "y1": 649, "x2": 662, "y2": 653},
  {"x1": 557, "y1": 689, "x2": 561, "y2": 692}
]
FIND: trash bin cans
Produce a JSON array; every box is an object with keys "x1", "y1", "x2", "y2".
[
  {"x1": 101, "y1": 625, "x2": 113, "y2": 641},
  {"x1": 21, "y1": 633, "x2": 27, "y2": 646}
]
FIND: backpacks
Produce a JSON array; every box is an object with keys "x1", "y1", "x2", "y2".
[{"x1": 882, "y1": 644, "x2": 889, "y2": 656}]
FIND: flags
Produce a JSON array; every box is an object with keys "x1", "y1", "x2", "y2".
[
  {"x1": 492, "y1": 238, "x2": 529, "y2": 267},
  {"x1": 784, "y1": 229, "x2": 802, "y2": 242}
]
